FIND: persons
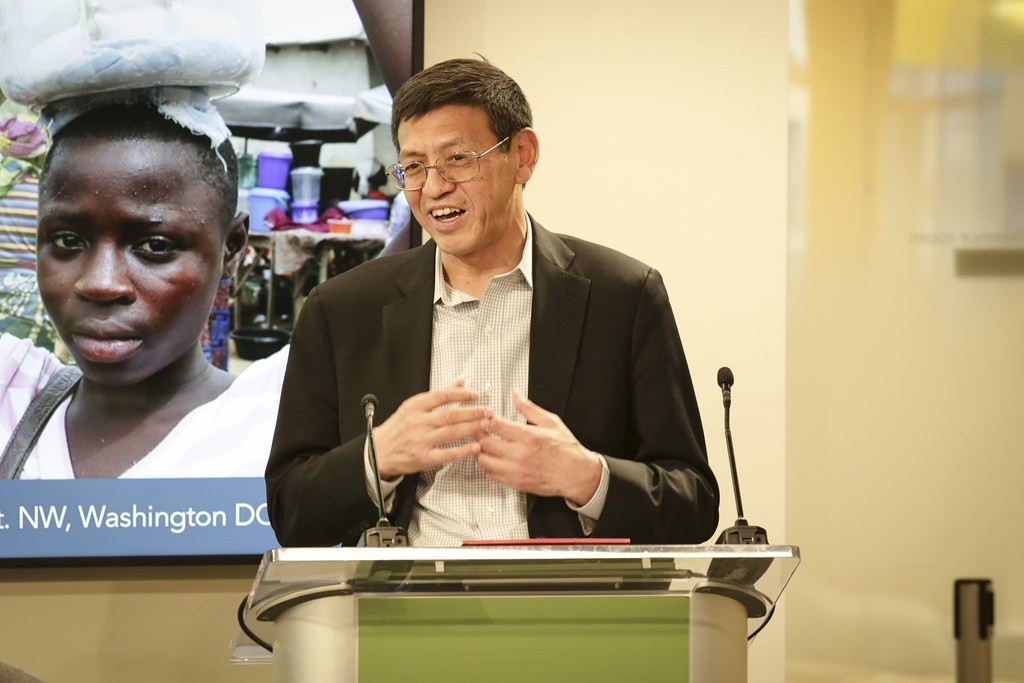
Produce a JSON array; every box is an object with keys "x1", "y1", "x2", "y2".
[
  {"x1": 365, "y1": 175, "x2": 390, "y2": 199},
  {"x1": 263, "y1": 53, "x2": 720, "y2": 546},
  {"x1": 0, "y1": 100, "x2": 411, "y2": 479}
]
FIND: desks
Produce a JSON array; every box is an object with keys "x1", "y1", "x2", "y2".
[{"x1": 225, "y1": 231, "x2": 385, "y2": 329}]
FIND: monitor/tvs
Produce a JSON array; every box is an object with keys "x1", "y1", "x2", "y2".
[{"x1": 0, "y1": 0, "x2": 424, "y2": 569}]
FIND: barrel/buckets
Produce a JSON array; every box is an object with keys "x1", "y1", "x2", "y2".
[{"x1": 248, "y1": 137, "x2": 325, "y2": 234}]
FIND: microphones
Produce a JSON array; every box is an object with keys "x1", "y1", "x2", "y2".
[
  {"x1": 702, "y1": 366, "x2": 774, "y2": 584},
  {"x1": 361, "y1": 394, "x2": 414, "y2": 582}
]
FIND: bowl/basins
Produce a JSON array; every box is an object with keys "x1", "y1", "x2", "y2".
[
  {"x1": 229, "y1": 328, "x2": 290, "y2": 361},
  {"x1": 346, "y1": 205, "x2": 388, "y2": 219}
]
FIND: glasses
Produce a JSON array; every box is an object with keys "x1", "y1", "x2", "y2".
[{"x1": 384, "y1": 134, "x2": 514, "y2": 192}]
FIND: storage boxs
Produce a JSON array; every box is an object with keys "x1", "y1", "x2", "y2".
[
  {"x1": 246, "y1": 139, "x2": 391, "y2": 232},
  {"x1": 203, "y1": 278, "x2": 233, "y2": 373}
]
